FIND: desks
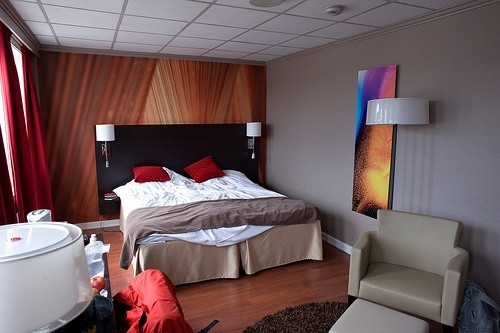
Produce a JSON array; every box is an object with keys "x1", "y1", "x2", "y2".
[{"x1": 82, "y1": 233, "x2": 115, "y2": 333}]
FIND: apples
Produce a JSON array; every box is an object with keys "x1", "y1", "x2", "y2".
[{"x1": 92, "y1": 276, "x2": 106, "y2": 294}]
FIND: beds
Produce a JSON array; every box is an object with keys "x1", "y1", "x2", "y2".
[{"x1": 94, "y1": 124, "x2": 323, "y2": 288}]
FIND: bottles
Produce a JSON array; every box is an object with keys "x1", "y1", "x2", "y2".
[{"x1": 85, "y1": 233, "x2": 105, "y2": 283}]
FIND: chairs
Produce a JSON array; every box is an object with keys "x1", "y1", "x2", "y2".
[{"x1": 347, "y1": 208, "x2": 470, "y2": 333}]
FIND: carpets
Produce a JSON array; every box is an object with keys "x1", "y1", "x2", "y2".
[{"x1": 243, "y1": 301, "x2": 351, "y2": 333}]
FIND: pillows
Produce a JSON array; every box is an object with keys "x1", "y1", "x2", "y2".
[
  {"x1": 183, "y1": 154, "x2": 226, "y2": 183},
  {"x1": 132, "y1": 165, "x2": 171, "y2": 183}
]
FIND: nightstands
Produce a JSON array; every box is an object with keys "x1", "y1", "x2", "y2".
[{"x1": 99, "y1": 197, "x2": 121, "y2": 214}]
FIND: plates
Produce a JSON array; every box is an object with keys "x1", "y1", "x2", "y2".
[{"x1": 95, "y1": 295, "x2": 114, "y2": 323}]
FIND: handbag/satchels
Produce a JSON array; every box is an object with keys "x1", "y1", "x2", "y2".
[{"x1": 458, "y1": 280, "x2": 500, "y2": 333}]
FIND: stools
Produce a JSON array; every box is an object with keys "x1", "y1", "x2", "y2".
[{"x1": 327, "y1": 299, "x2": 429, "y2": 333}]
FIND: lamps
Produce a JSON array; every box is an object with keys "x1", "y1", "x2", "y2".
[
  {"x1": 367, "y1": 97, "x2": 430, "y2": 210},
  {"x1": 96, "y1": 125, "x2": 115, "y2": 168},
  {"x1": 246, "y1": 121, "x2": 262, "y2": 159},
  {"x1": 0, "y1": 221, "x2": 93, "y2": 333}
]
